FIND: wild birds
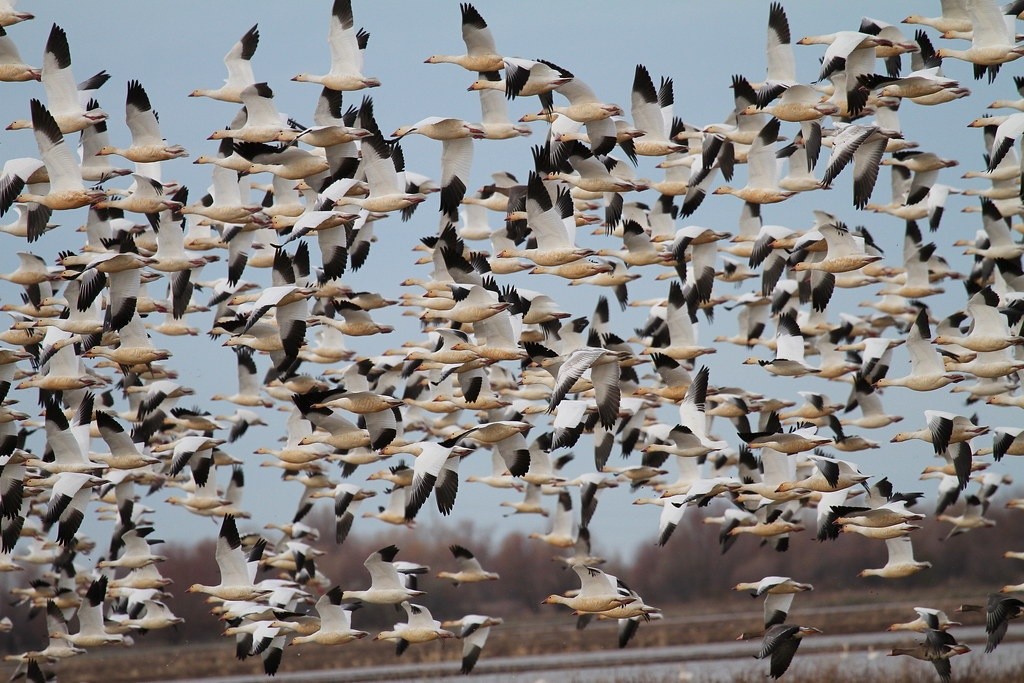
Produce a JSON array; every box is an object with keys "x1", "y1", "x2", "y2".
[{"x1": 0, "y1": 0, "x2": 1024, "y2": 682}]
